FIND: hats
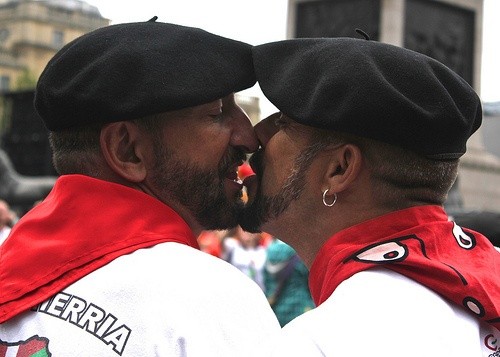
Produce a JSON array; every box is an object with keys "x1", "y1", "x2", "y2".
[
  {"x1": 253, "y1": 28, "x2": 483, "y2": 158},
  {"x1": 36, "y1": 15, "x2": 258, "y2": 130}
]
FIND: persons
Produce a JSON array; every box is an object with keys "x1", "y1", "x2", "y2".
[
  {"x1": 0, "y1": 87, "x2": 317, "y2": 327},
  {"x1": 238, "y1": 26, "x2": 499, "y2": 357},
  {"x1": 0, "y1": 14, "x2": 286, "y2": 357}
]
